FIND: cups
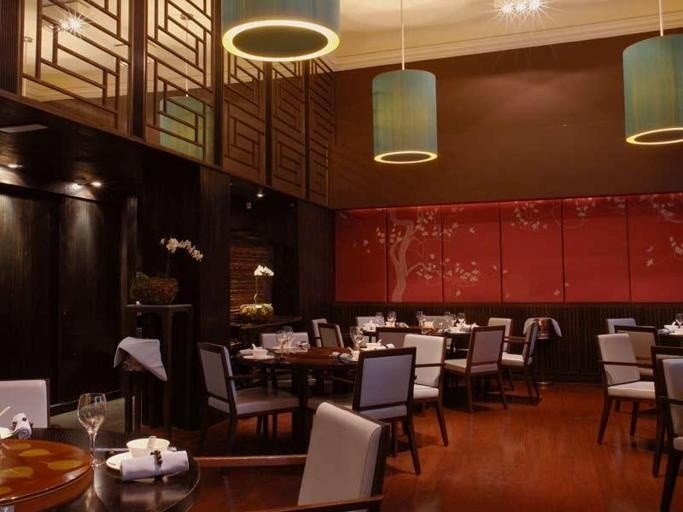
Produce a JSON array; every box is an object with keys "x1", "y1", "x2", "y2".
[
  {"x1": 350, "y1": 325, "x2": 364, "y2": 351},
  {"x1": 416, "y1": 311, "x2": 423, "y2": 329},
  {"x1": 77, "y1": 393, "x2": 107, "y2": 466},
  {"x1": 444, "y1": 312, "x2": 451, "y2": 322},
  {"x1": 450, "y1": 314, "x2": 457, "y2": 327},
  {"x1": 676, "y1": 313, "x2": 683, "y2": 328},
  {"x1": 457, "y1": 313, "x2": 464, "y2": 331},
  {"x1": 276, "y1": 330, "x2": 287, "y2": 360},
  {"x1": 284, "y1": 326, "x2": 293, "y2": 355}
]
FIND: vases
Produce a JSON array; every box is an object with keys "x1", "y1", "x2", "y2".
[
  {"x1": 133, "y1": 276, "x2": 182, "y2": 306},
  {"x1": 242, "y1": 303, "x2": 274, "y2": 323}
]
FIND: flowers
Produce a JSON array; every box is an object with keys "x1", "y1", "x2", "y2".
[
  {"x1": 253, "y1": 264, "x2": 274, "y2": 305},
  {"x1": 161, "y1": 237, "x2": 203, "y2": 276}
]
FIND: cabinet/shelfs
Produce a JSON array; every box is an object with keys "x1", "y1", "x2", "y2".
[
  {"x1": 274, "y1": 201, "x2": 336, "y2": 326},
  {"x1": 233, "y1": 315, "x2": 306, "y2": 343},
  {"x1": 125, "y1": 304, "x2": 194, "y2": 436},
  {"x1": 137, "y1": 164, "x2": 229, "y2": 437}
]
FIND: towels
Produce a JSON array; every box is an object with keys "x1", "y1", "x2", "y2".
[
  {"x1": 462, "y1": 322, "x2": 479, "y2": 331},
  {"x1": 375, "y1": 343, "x2": 394, "y2": 350},
  {"x1": 11, "y1": 412, "x2": 32, "y2": 440},
  {"x1": 523, "y1": 316, "x2": 561, "y2": 337},
  {"x1": 331, "y1": 351, "x2": 351, "y2": 361},
  {"x1": 119, "y1": 450, "x2": 189, "y2": 482},
  {"x1": 114, "y1": 336, "x2": 167, "y2": 382},
  {"x1": 296, "y1": 341, "x2": 310, "y2": 350}
]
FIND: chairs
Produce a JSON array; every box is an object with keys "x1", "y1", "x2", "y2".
[
  {"x1": 488, "y1": 318, "x2": 515, "y2": 391},
  {"x1": 420, "y1": 315, "x2": 446, "y2": 330},
  {"x1": 660, "y1": 357, "x2": 683, "y2": 512},
  {"x1": 316, "y1": 322, "x2": 346, "y2": 347},
  {"x1": 197, "y1": 401, "x2": 390, "y2": 512},
  {"x1": 261, "y1": 332, "x2": 312, "y2": 348},
  {"x1": 502, "y1": 324, "x2": 540, "y2": 398},
  {"x1": 616, "y1": 323, "x2": 660, "y2": 361},
  {"x1": 196, "y1": 342, "x2": 305, "y2": 452},
  {"x1": 444, "y1": 326, "x2": 508, "y2": 414},
  {"x1": 607, "y1": 318, "x2": 635, "y2": 338},
  {"x1": 535, "y1": 319, "x2": 558, "y2": 383},
  {"x1": 374, "y1": 326, "x2": 419, "y2": 350},
  {"x1": 311, "y1": 318, "x2": 327, "y2": 348},
  {"x1": 598, "y1": 334, "x2": 664, "y2": 442},
  {"x1": 309, "y1": 347, "x2": 421, "y2": 477},
  {"x1": 0, "y1": 380, "x2": 53, "y2": 430},
  {"x1": 356, "y1": 316, "x2": 385, "y2": 341},
  {"x1": 651, "y1": 343, "x2": 683, "y2": 475},
  {"x1": 404, "y1": 334, "x2": 449, "y2": 448}
]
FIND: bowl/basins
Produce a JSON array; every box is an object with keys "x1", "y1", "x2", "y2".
[
  {"x1": 250, "y1": 349, "x2": 268, "y2": 356},
  {"x1": 127, "y1": 438, "x2": 170, "y2": 457},
  {"x1": 664, "y1": 324, "x2": 678, "y2": 332},
  {"x1": 366, "y1": 343, "x2": 381, "y2": 349}
]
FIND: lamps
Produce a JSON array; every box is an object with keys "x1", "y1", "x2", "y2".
[
  {"x1": 59, "y1": 17, "x2": 87, "y2": 37},
  {"x1": 72, "y1": 183, "x2": 83, "y2": 190},
  {"x1": 257, "y1": 193, "x2": 265, "y2": 198},
  {"x1": 221, "y1": 1, "x2": 340, "y2": 62},
  {"x1": 8, "y1": 163, "x2": 19, "y2": 169},
  {"x1": 492, "y1": 1, "x2": 545, "y2": 15},
  {"x1": 372, "y1": 4, "x2": 437, "y2": 164},
  {"x1": 624, "y1": 0, "x2": 683, "y2": 146},
  {"x1": 92, "y1": 181, "x2": 102, "y2": 189}
]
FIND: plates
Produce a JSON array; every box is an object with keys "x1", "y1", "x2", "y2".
[
  {"x1": 243, "y1": 355, "x2": 274, "y2": 360},
  {"x1": 106, "y1": 452, "x2": 132, "y2": 471}
]
